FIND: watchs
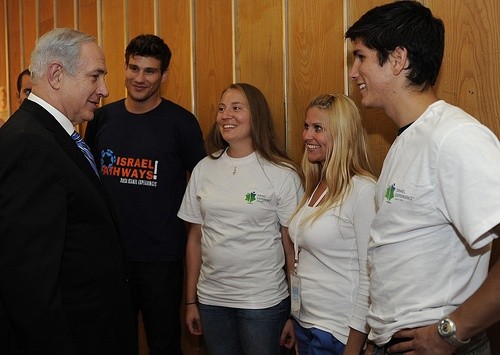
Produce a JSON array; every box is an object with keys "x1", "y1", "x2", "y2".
[{"x1": 437, "y1": 318, "x2": 471, "y2": 347}]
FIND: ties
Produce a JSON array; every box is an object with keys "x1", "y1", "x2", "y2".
[{"x1": 72, "y1": 131, "x2": 99, "y2": 179}]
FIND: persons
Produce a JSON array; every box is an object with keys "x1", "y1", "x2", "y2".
[
  {"x1": 0, "y1": 28, "x2": 140, "y2": 355},
  {"x1": 342, "y1": 0, "x2": 500, "y2": 355},
  {"x1": 84, "y1": 34, "x2": 208, "y2": 355},
  {"x1": 286, "y1": 92, "x2": 378, "y2": 355},
  {"x1": 177, "y1": 83, "x2": 304, "y2": 355}
]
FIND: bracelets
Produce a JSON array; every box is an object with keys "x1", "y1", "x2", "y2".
[{"x1": 185, "y1": 302, "x2": 196, "y2": 305}]
